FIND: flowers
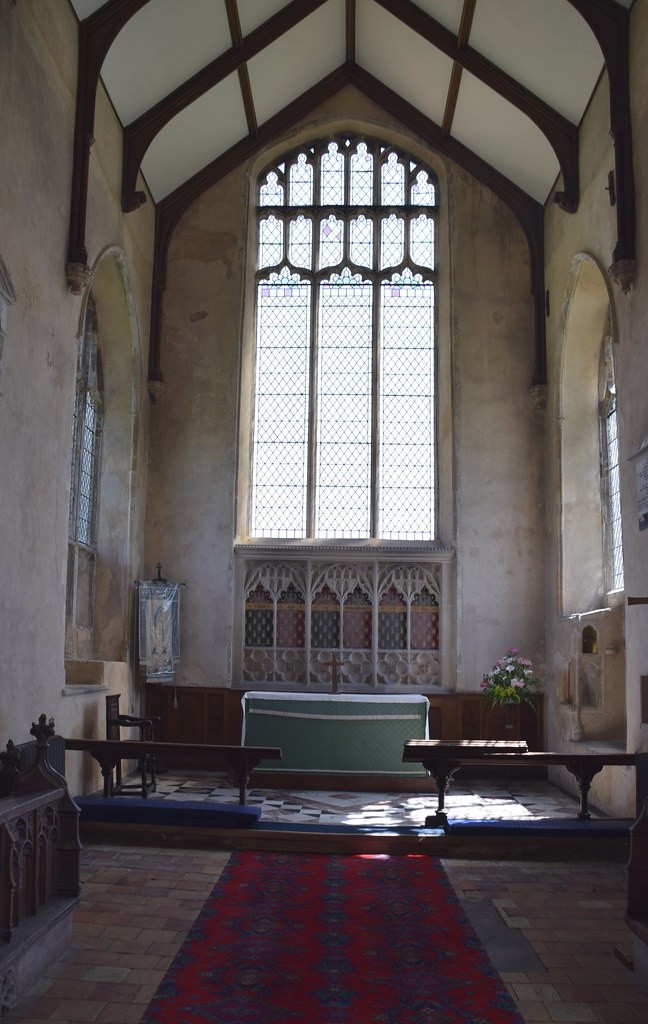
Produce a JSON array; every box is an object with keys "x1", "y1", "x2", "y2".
[{"x1": 476, "y1": 646, "x2": 541, "y2": 710}]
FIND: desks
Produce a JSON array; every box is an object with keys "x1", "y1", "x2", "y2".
[{"x1": 241, "y1": 692, "x2": 431, "y2": 778}]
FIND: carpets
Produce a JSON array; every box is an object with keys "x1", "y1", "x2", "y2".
[{"x1": 139, "y1": 847, "x2": 524, "y2": 1024}]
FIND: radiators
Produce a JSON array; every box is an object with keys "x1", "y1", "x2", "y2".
[{"x1": 15, "y1": 736, "x2": 65, "y2": 779}]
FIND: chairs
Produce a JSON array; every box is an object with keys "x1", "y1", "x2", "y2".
[{"x1": 105, "y1": 692, "x2": 161, "y2": 798}]
